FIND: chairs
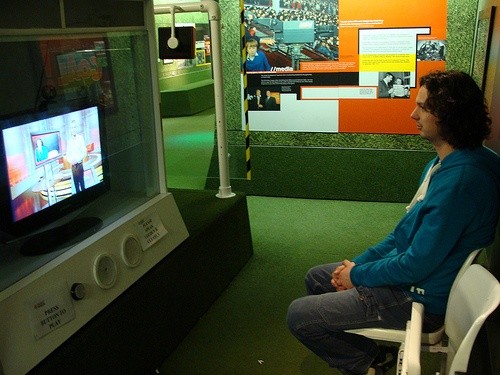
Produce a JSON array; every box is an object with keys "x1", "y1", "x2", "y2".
[
  {"x1": 342, "y1": 247, "x2": 483, "y2": 346},
  {"x1": 398, "y1": 263, "x2": 500, "y2": 375}
]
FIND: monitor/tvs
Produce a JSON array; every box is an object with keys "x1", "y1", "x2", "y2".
[{"x1": 0, "y1": 103, "x2": 111, "y2": 255}]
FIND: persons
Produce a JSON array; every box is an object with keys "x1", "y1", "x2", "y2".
[
  {"x1": 247, "y1": 90, "x2": 276, "y2": 111},
  {"x1": 288, "y1": 71, "x2": 500, "y2": 375},
  {"x1": 35, "y1": 120, "x2": 86, "y2": 195},
  {"x1": 247, "y1": 40, "x2": 271, "y2": 72},
  {"x1": 379, "y1": 72, "x2": 410, "y2": 98}
]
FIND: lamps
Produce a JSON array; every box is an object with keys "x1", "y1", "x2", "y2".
[{"x1": 168, "y1": 5, "x2": 179, "y2": 49}]
